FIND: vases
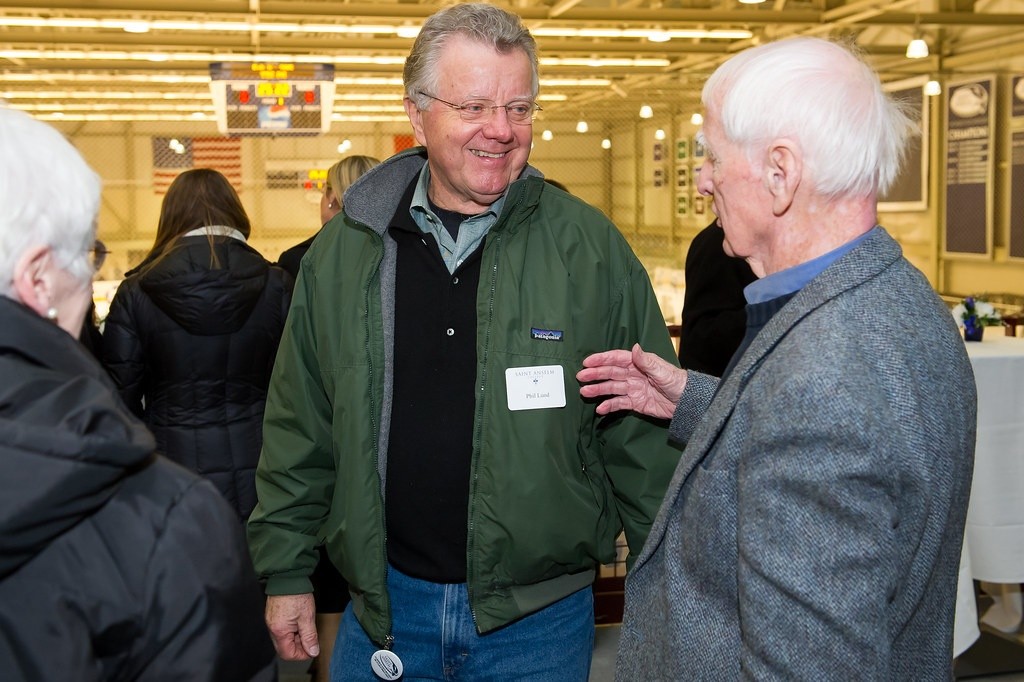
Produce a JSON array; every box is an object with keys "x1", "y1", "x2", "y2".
[{"x1": 963, "y1": 326, "x2": 985, "y2": 343}]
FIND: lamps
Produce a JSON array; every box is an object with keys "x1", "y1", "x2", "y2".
[{"x1": 905, "y1": 30, "x2": 929, "y2": 58}]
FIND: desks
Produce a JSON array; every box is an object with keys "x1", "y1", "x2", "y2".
[{"x1": 953, "y1": 336, "x2": 1024, "y2": 660}]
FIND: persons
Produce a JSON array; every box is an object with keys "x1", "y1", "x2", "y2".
[
  {"x1": 678, "y1": 217, "x2": 758, "y2": 378},
  {"x1": 0, "y1": 106, "x2": 246, "y2": 682},
  {"x1": 577, "y1": 35, "x2": 979, "y2": 682},
  {"x1": 246, "y1": 3, "x2": 685, "y2": 682},
  {"x1": 101, "y1": 167, "x2": 294, "y2": 682},
  {"x1": 278, "y1": 154, "x2": 382, "y2": 274}
]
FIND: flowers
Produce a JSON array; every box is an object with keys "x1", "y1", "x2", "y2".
[{"x1": 951, "y1": 290, "x2": 1010, "y2": 334}]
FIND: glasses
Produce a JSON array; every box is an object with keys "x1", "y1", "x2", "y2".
[
  {"x1": 417, "y1": 90, "x2": 543, "y2": 126},
  {"x1": 83, "y1": 240, "x2": 110, "y2": 270}
]
